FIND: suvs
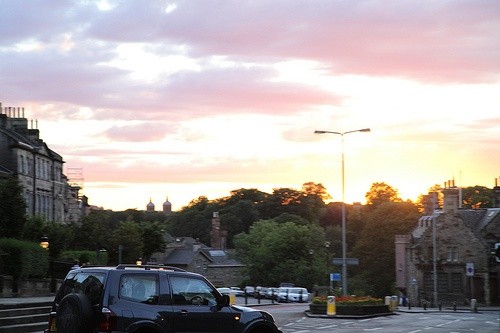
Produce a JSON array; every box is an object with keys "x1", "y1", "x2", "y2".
[{"x1": 43, "y1": 261, "x2": 283, "y2": 333}]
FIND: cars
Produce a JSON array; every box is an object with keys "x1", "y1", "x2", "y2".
[{"x1": 216, "y1": 283, "x2": 311, "y2": 303}]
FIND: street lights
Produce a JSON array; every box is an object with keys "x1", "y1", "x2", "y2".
[
  {"x1": 433, "y1": 187, "x2": 459, "y2": 307},
  {"x1": 39, "y1": 234, "x2": 56, "y2": 293},
  {"x1": 324, "y1": 240, "x2": 331, "y2": 286},
  {"x1": 314, "y1": 129, "x2": 372, "y2": 298},
  {"x1": 309, "y1": 249, "x2": 315, "y2": 293},
  {"x1": 96, "y1": 246, "x2": 108, "y2": 265}
]
